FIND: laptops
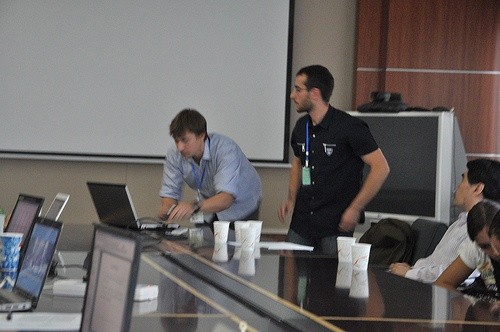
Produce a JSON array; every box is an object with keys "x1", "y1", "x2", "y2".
[
  {"x1": 85, "y1": 182, "x2": 179, "y2": 229},
  {"x1": 0, "y1": 217, "x2": 65, "y2": 312},
  {"x1": 44, "y1": 192, "x2": 71, "y2": 221},
  {"x1": 4, "y1": 193, "x2": 45, "y2": 250}
]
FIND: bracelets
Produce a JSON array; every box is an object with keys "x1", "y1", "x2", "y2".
[{"x1": 193, "y1": 201, "x2": 201, "y2": 214}]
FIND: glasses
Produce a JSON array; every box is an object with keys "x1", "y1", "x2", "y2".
[{"x1": 292, "y1": 87, "x2": 308, "y2": 94}]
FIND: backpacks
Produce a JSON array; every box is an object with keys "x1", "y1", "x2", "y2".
[{"x1": 360, "y1": 218, "x2": 417, "y2": 268}]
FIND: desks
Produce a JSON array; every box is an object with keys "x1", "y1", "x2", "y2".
[{"x1": 0, "y1": 221, "x2": 500, "y2": 332}]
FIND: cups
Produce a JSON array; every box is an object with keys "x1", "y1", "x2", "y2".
[
  {"x1": 212, "y1": 244, "x2": 228, "y2": 262},
  {"x1": 349, "y1": 270, "x2": 369, "y2": 299},
  {"x1": 0, "y1": 271, "x2": 18, "y2": 290},
  {"x1": 238, "y1": 251, "x2": 255, "y2": 276},
  {"x1": 0, "y1": 232, "x2": 23, "y2": 271},
  {"x1": 234, "y1": 220, "x2": 263, "y2": 251},
  {"x1": 336, "y1": 236, "x2": 356, "y2": 263},
  {"x1": 233, "y1": 247, "x2": 241, "y2": 260},
  {"x1": 213, "y1": 221, "x2": 230, "y2": 244},
  {"x1": 351, "y1": 243, "x2": 371, "y2": 270},
  {"x1": 254, "y1": 249, "x2": 261, "y2": 259},
  {"x1": 335, "y1": 263, "x2": 352, "y2": 289}
]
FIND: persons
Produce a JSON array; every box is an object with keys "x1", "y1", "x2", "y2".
[
  {"x1": 433, "y1": 199, "x2": 500, "y2": 301},
  {"x1": 277, "y1": 65, "x2": 389, "y2": 254},
  {"x1": 385, "y1": 159, "x2": 500, "y2": 291},
  {"x1": 160, "y1": 109, "x2": 263, "y2": 231}
]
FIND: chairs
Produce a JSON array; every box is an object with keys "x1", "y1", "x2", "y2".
[{"x1": 411, "y1": 220, "x2": 448, "y2": 265}]
